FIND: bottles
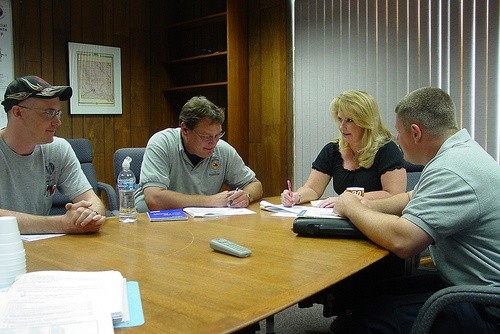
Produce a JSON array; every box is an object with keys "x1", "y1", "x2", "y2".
[{"x1": 117, "y1": 156, "x2": 137, "y2": 223}]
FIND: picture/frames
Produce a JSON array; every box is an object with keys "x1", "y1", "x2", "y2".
[{"x1": 65, "y1": 40, "x2": 123, "y2": 116}]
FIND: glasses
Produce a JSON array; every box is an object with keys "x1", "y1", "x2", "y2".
[
  {"x1": 17, "y1": 104, "x2": 63, "y2": 119},
  {"x1": 194, "y1": 130, "x2": 225, "y2": 140}
]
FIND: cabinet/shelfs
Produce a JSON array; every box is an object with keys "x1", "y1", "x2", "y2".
[{"x1": 161, "y1": 0, "x2": 250, "y2": 166}]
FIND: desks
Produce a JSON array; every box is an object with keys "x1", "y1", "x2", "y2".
[{"x1": 19, "y1": 195, "x2": 392, "y2": 334}]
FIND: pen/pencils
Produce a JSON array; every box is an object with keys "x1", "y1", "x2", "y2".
[
  {"x1": 228, "y1": 187, "x2": 238, "y2": 207},
  {"x1": 287, "y1": 180, "x2": 293, "y2": 208}
]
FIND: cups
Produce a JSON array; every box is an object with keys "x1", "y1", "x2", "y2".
[
  {"x1": 346, "y1": 187, "x2": 364, "y2": 199},
  {"x1": 0, "y1": 216, "x2": 27, "y2": 288}
]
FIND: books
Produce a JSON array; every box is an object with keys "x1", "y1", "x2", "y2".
[{"x1": 146, "y1": 209, "x2": 188, "y2": 221}]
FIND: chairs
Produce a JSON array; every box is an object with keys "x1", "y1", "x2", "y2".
[
  {"x1": 113, "y1": 147, "x2": 145, "y2": 188},
  {"x1": 47, "y1": 138, "x2": 119, "y2": 216}
]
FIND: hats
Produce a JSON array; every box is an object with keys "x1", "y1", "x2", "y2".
[{"x1": 1, "y1": 75, "x2": 72, "y2": 113}]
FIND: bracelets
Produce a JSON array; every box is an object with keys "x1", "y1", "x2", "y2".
[{"x1": 246, "y1": 192, "x2": 251, "y2": 204}]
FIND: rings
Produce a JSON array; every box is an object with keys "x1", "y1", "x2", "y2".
[{"x1": 93, "y1": 210, "x2": 99, "y2": 215}]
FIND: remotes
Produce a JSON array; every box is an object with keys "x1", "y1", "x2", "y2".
[{"x1": 210, "y1": 239, "x2": 251, "y2": 258}]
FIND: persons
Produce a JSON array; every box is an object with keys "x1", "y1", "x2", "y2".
[
  {"x1": 0, "y1": 76, "x2": 106, "y2": 233},
  {"x1": 135, "y1": 96, "x2": 263, "y2": 212},
  {"x1": 331, "y1": 86, "x2": 500, "y2": 334},
  {"x1": 281, "y1": 90, "x2": 407, "y2": 317}
]
